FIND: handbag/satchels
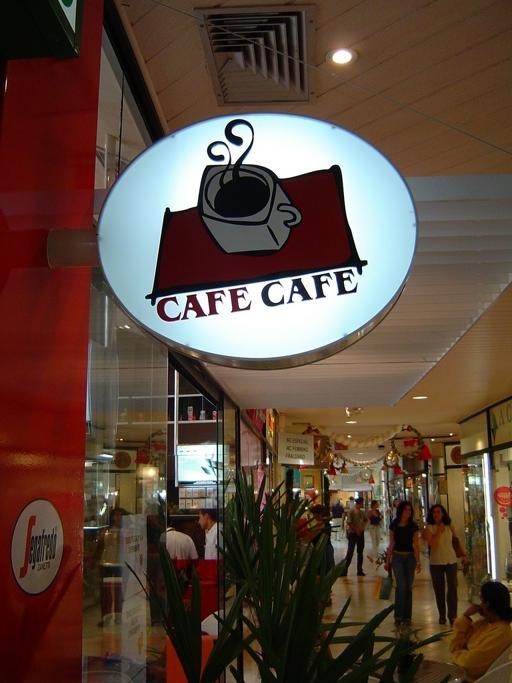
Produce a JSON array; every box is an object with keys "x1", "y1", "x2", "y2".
[
  {"x1": 452, "y1": 537, "x2": 466, "y2": 558},
  {"x1": 373, "y1": 577, "x2": 393, "y2": 599}
]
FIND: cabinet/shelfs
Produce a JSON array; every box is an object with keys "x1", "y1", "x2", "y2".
[{"x1": 113, "y1": 391, "x2": 223, "y2": 426}]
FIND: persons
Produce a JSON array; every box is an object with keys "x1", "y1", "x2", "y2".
[
  {"x1": 198, "y1": 509, "x2": 233, "y2": 560},
  {"x1": 448, "y1": 581, "x2": 512, "y2": 683},
  {"x1": 159, "y1": 516, "x2": 199, "y2": 560},
  {"x1": 332, "y1": 496, "x2": 401, "y2": 576},
  {"x1": 422, "y1": 504, "x2": 468, "y2": 625},
  {"x1": 88, "y1": 508, "x2": 129, "y2": 627},
  {"x1": 384, "y1": 501, "x2": 422, "y2": 627}
]
